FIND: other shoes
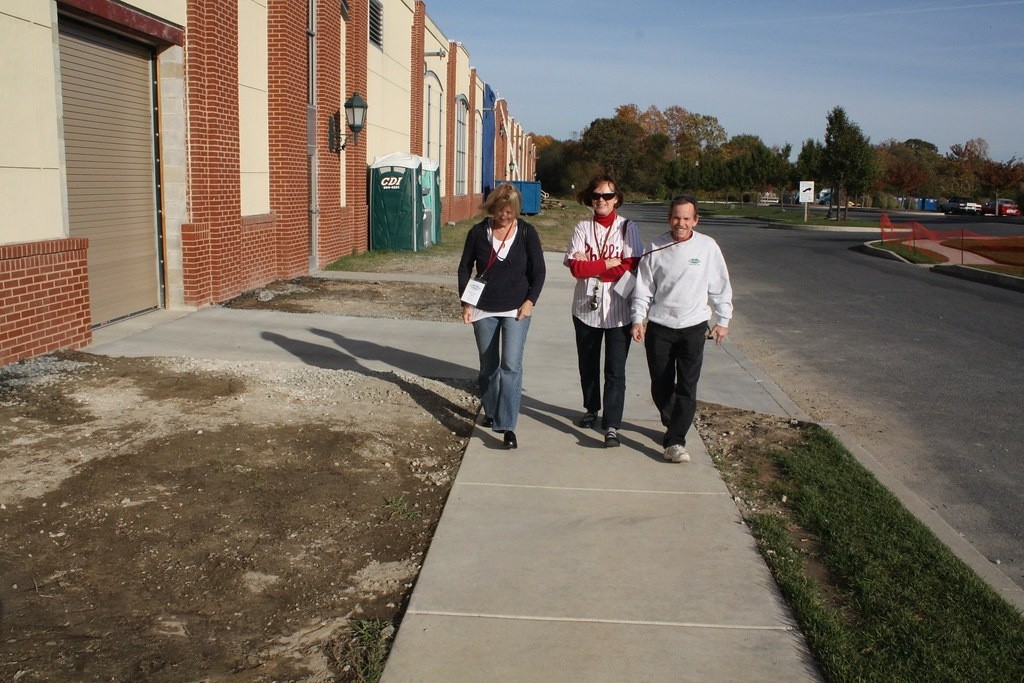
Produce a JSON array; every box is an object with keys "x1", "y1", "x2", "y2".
[
  {"x1": 581, "y1": 411, "x2": 600, "y2": 428},
  {"x1": 504, "y1": 431, "x2": 519, "y2": 448},
  {"x1": 605, "y1": 432, "x2": 620, "y2": 446},
  {"x1": 483, "y1": 415, "x2": 493, "y2": 426}
]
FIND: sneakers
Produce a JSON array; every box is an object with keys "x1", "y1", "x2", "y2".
[{"x1": 664, "y1": 444, "x2": 691, "y2": 462}]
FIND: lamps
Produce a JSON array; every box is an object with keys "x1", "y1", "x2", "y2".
[{"x1": 328, "y1": 92, "x2": 369, "y2": 152}]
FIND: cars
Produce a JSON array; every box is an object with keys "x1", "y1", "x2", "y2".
[
  {"x1": 982, "y1": 198, "x2": 1021, "y2": 218},
  {"x1": 785, "y1": 187, "x2": 832, "y2": 205},
  {"x1": 938, "y1": 196, "x2": 982, "y2": 217}
]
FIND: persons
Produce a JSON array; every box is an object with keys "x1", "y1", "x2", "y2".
[
  {"x1": 458, "y1": 185, "x2": 545, "y2": 448},
  {"x1": 564, "y1": 175, "x2": 643, "y2": 447},
  {"x1": 630, "y1": 195, "x2": 733, "y2": 462}
]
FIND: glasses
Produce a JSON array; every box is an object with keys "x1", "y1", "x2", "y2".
[{"x1": 591, "y1": 192, "x2": 616, "y2": 201}]
